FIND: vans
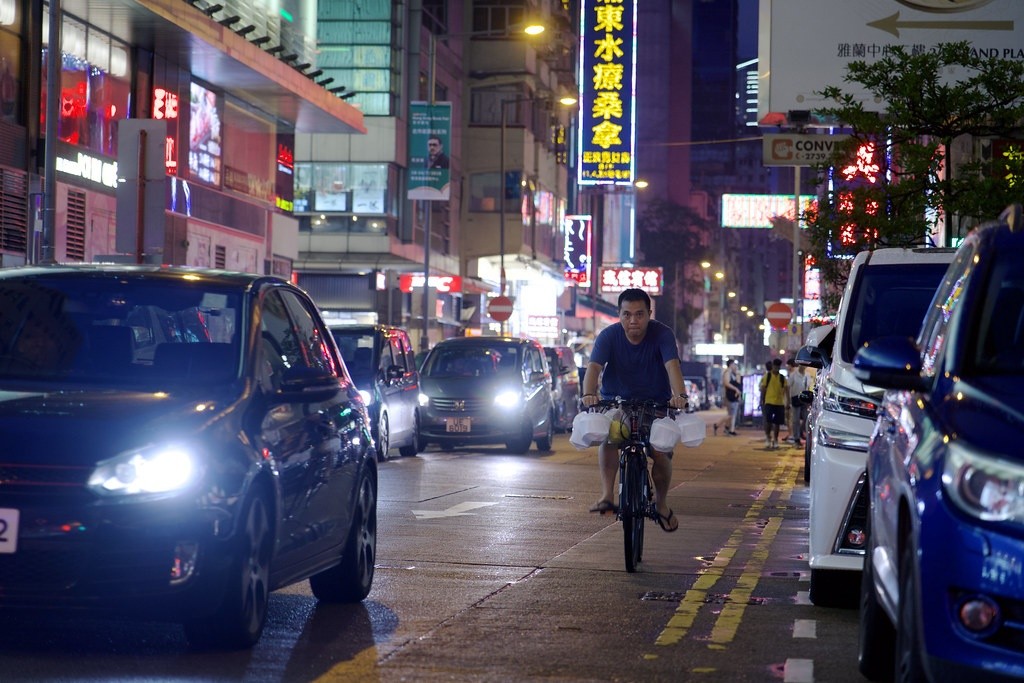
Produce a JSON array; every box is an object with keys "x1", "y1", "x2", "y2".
[{"x1": 326, "y1": 323, "x2": 422, "y2": 463}]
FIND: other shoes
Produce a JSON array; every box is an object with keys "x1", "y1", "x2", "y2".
[
  {"x1": 795, "y1": 439, "x2": 802, "y2": 447},
  {"x1": 728, "y1": 431, "x2": 737, "y2": 436},
  {"x1": 800, "y1": 432, "x2": 806, "y2": 440},
  {"x1": 782, "y1": 435, "x2": 789, "y2": 441},
  {"x1": 724, "y1": 429, "x2": 729, "y2": 434},
  {"x1": 772, "y1": 440, "x2": 778, "y2": 448},
  {"x1": 713, "y1": 423, "x2": 718, "y2": 436},
  {"x1": 766, "y1": 436, "x2": 772, "y2": 448}
]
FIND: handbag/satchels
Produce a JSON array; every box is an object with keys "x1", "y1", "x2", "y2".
[
  {"x1": 725, "y1": 379, "x2": 742, "y2": 402},
  {"x1": 791, "y1": 395, "x2": 802, "y2": 407}
]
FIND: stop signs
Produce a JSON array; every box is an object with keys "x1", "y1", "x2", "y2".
[
  {"x1": 767, "y1": 303, "x2": 792, "y2": 327},
  {"x1": 501, "y1": 270, "x2": 506, "y2": 291},
  {"x1": 488, "y1": 296, "x2": 513, "y2": 322}
]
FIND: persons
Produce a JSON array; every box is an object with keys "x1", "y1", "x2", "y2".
[
  {"x1": 581, "y1": 288, "x2": 689, "y2": 533},
  {"x1": 424, "y1": 135, "x2": 449, "y2": 169},
  {"x1": 712, "y1": 359, "x2": 742, "y2": 436},
  {"x1": 759, "y1": 358, "x2": 813, "y2": 450}
]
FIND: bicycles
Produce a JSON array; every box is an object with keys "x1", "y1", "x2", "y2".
[{"x1": 584, "y1": 397, "x2": 689, "y2": 572}]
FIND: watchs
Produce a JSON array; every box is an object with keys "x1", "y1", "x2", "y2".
[{"x1": 675, "y1": 392, "x2": 689, "y2": 401}]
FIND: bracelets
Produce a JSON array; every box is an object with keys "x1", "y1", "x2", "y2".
[{"x1": 581, "y1": 393, "x2": 595, "y2": 398}]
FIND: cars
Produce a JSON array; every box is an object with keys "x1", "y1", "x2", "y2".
[
  {"x1": 418, "y1": 336, "x2": 555, "y2": 453},
  {"x1": 543, "y1": 346, "x2": 580, "y2": 432}
]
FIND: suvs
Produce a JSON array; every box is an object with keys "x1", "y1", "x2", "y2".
[
  {"x1": 0, "y1": 263, "x2": 377, "y2": 651},
  {"x1": 794, "y1": 200, "x2": 1024, "y2": 683}
]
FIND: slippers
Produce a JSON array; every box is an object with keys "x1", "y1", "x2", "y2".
[
  {"x1": 589, "y1": 499, "x2": 615, "y2": 512},
  {"x1": 654, "y1": 504, "x2": 678, "y2": 533}
]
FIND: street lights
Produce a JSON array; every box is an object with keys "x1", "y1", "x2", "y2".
[
  {"x1": 422, "y1": 21, "x2": 545, "y2": 346},
  {"x1": 499, "y1": 96, "x2": 578, "y2": 335},
  {"x1": 591, "y1": 181, "x2": 649, "y2": 341}
]
